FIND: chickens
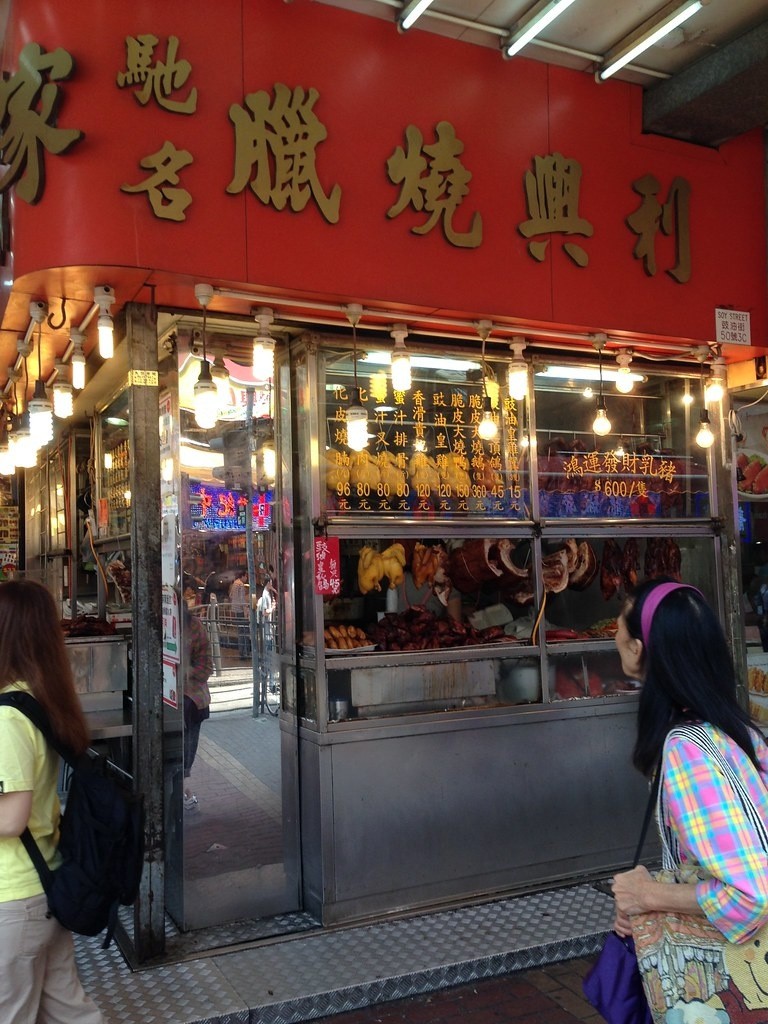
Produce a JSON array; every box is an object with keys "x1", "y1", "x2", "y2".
[
  {"x1": 324, "y1": 445, "x2": 499, "y2": 500},
  {"x1": 358, "y1": 547, "x2": 383, "y2": 594},
  {"x1": 381, "y1": 543, "x2": 405, "y2": 590},
  {"x1": 413, "y1": 541, "x2": 454, "y2": 607}
]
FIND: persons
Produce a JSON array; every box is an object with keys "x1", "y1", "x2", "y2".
[
  {"x1": 230, "y1": 565, "x2": 278, "y2": 692},
  {"x1": 0, "y1": 578, "x2": 106, "y2": 1024},
  {"x1": 610, "y1": 577, "x2": 768, "y2": 1024},
  {"x1": 151, "y1": 587, "x2": 214, "y2": 815}
]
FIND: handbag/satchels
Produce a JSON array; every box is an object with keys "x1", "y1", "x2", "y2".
[{"x1": 582, "y1": 722, "x2": 768, "y2": 1024}]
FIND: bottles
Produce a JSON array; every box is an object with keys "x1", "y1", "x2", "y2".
[{"x1": 329, "y1": 696, "x2": 349, "y2": 720}]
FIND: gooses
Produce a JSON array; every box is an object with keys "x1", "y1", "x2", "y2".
[{"x1": 521, "y1": 434, "x2": 680, "y2": 496}]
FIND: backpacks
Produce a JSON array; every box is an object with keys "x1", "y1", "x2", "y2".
[{"x1": 0, "y1": 692, "x2": 145, "y2": 951}]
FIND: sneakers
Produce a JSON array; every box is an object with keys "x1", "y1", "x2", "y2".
[{"x1": 183, "y1": 792, "x2": 201, "y2": 816}]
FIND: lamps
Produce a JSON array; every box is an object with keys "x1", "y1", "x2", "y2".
[
  {"x1": 0, "y1": 286, "x2": 116, "y2": 475},
  {"x1": 194, "y1": 284, "x2": 728, "y2": 447}
]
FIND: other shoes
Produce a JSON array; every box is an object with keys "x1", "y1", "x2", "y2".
[{"x1": 237, "y1": 652, "x2": 253, "y2": 661}]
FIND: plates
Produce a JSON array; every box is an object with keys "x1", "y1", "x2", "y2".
[
  {"x1": 749, "y1": 687, "x2": 768, "y2": 696},
  {"x1": 735, "y1": 448, "x2": 768, "y2": 499},
  {"x1": 751, "y1": 702, "x2": 768, "y2": 727}
]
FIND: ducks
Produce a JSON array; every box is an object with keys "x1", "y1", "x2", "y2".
[{"x1": 600, "y1": 536, "x2": 681, "y2": 605}]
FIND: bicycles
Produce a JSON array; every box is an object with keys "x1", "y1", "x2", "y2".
[{"x1": 263, "y1": 665, "x2": 281, "y2": 716}]
